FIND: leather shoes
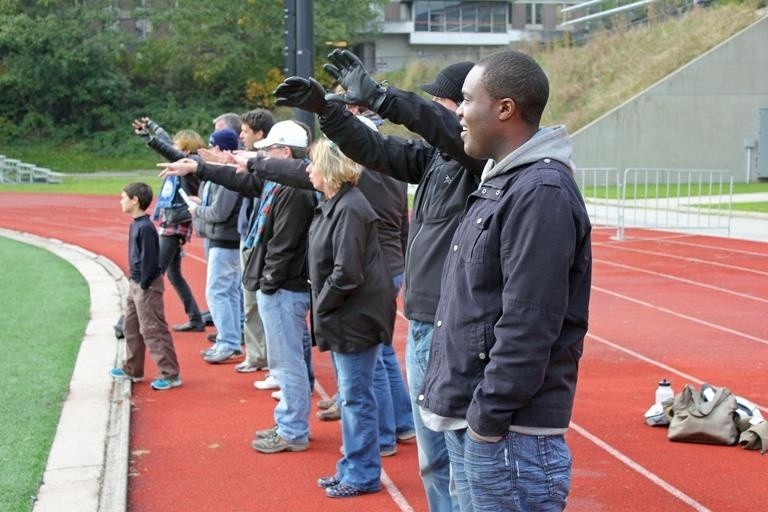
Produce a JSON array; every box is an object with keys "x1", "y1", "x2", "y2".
[{"x1": 172, "y1": 320, "x2": 204, "y2": 332}]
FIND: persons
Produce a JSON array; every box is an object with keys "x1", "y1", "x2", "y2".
[
  {"x1": 110, "y1": 182, "x2": 185, "y2": 390},
  {"x1": 133, "y1": 81, "x2": 415, "y2": 496},
  {"x1": 272, "y1": 48, "x2": 477, "y2": 508},
  {"x1": 320, "y1": 46, "x2": 594, "y2": 508}
]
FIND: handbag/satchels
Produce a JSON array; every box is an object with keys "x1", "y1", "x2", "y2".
[
  {"x1": 667, "y1": 384, "x2": 738, "y2": 446},
  {"x1": 704, "y1": 387, "x2": 763, "y2": 432}
]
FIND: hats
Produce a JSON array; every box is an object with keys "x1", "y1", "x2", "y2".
[
  {"x1": 209, "y1": 128, "x2": 238, "y2": 150},
  {"x1": 421, "y1": 62, "x2": 475, "y2": 101},
  {"x1": 253, "y1": 120, "x2": 308, "y2": 149}
]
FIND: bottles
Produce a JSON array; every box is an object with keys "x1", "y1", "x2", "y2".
[{"x1": 654, "y1": 379, "x2": 673, "y2": 404}]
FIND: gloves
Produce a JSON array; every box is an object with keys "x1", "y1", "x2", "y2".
[
  {"x1": 322, "y1": 48, "x2": 386, "y2": 109},
  {"x1": 272, "y1": 76, "x2": 342, "y2": 124}
]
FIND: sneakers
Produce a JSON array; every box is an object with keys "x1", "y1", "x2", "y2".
[
  {"x1": 111, "y1": 368, "x2": 144, "y2": 381},
  {"x1": 151, "y1": 378, "x2": 181, "y2": 390}
]
